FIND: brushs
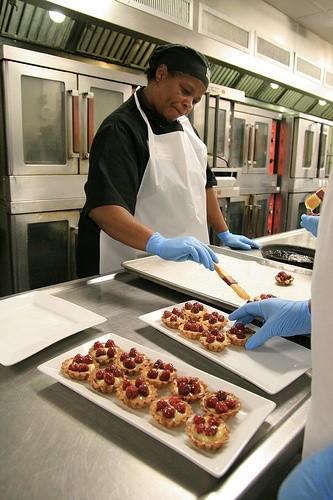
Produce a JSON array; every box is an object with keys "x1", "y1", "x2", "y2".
[
  {"x1": 213, "y1": 261, "x2": 250, "y2": 300},
  {"x1": 305, "y1": 187, "x2": 326, "y2": 210}
]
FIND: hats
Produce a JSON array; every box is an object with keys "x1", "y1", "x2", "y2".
[{"x1": 149, "y1": 43, "x2": 211, "y2": 89}]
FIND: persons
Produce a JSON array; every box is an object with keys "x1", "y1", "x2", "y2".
[
  {"x1": 76, "y1": 44, "x2": 260, "y2": 279},
  {"x1": 229, "y1": 161, "x2": 333, "y2": 500}
]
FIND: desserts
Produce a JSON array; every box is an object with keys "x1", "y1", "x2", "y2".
[
  {"x1": 162, "y1": 302, "x2": 255, "y2": 351},
  {"x1": 246, "y1": 293, "x2": 276, "y2": 303},
  {"x1": 275, "y1": 271, "x2": 294, "y2": 285},
  {"x1": 61, "y1": 338, "x2": 243, "y2": 451}
]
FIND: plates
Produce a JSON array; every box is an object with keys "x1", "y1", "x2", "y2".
[
  {"x1": 259, "y1": 242, "x2": 316, "y2": 269},
  {"x1": 138, "y1": 300, "x2": 313, "y2": 395},
  {"x1": 0, "y1": 290, "x2": 107, "y2": 366},
  {"x1": 37, "y1": 332, "x2": 276, "y2": 478}
]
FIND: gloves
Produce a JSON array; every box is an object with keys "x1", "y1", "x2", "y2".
[
  {"x1": 300, "y1": 214, "x2": 320, "y2": 238},
  {"x1": 145, "y1": 231, "x2": 219, "y2": 271},
  {"x1": 229, "y1": 298, "x2": 311, "y2": 349},
  {"x1": 216, "y1": 229, "x2": 261, "y2": 250}
]
